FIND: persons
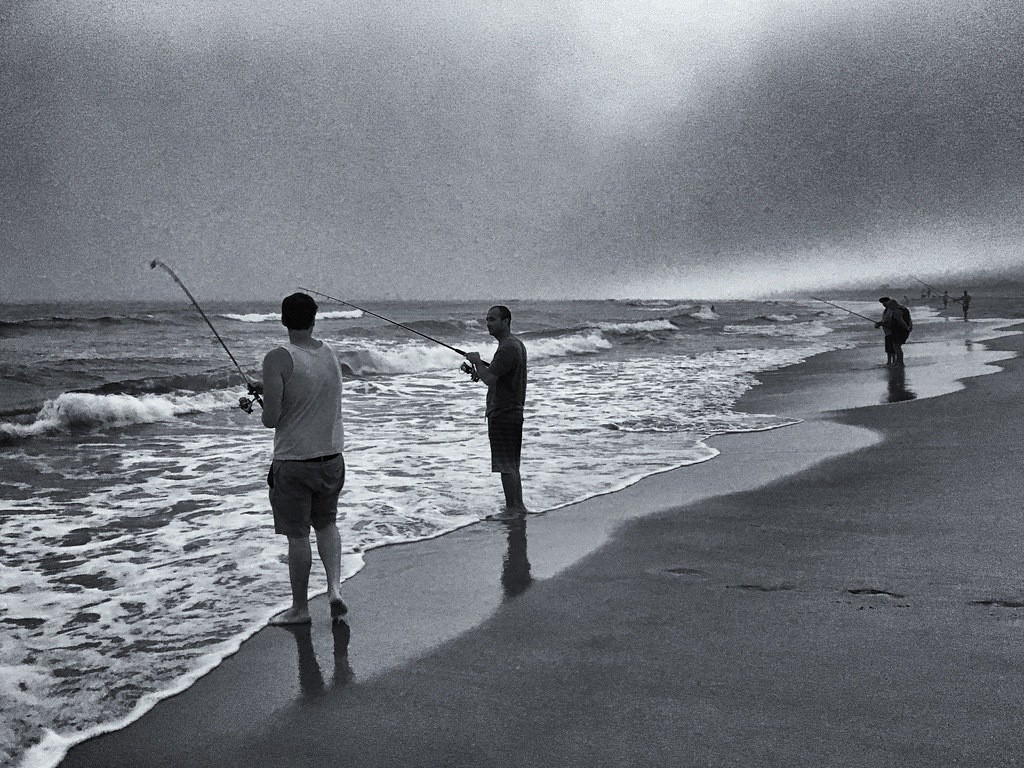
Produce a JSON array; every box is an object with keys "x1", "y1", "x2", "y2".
[
  {"x1": 466, "y1": 306, "x2": 527, "y2": 521},
  {"x1": 247, "y1": 293, "x2": 349, "y2": 623},
  {"x1": 874, "y1": 297, "x2": 912, "y2": 365},
  {"x1": 943, "y1": 291, "x2": 949, "y2": 309},
  {"x1": 957, "y1": 291, "x2": 971, "y2": 321}
]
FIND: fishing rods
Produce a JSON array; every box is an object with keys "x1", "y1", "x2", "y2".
[
  {"x1": 910, "y1": 274, "x2": 963, "y2": 305},
  {"x1": 149, "y1": 256, "x2": 267, "y2": 415},
  {"x1": 297, "y1": 285, "x2": 492, "y2": 382},
  {"x1": 810, "y1": 295, "x2": 881, "y2": 330}
]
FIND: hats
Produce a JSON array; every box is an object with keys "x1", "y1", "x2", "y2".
[{"x1": 880, "y1": 297, "x2": 890, "y2": 302}]
